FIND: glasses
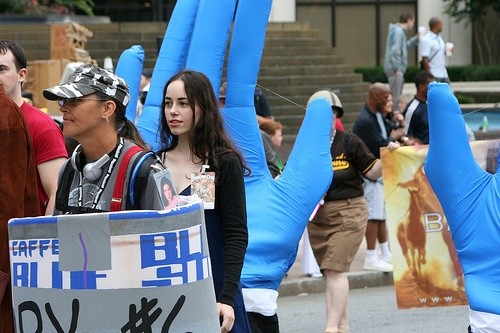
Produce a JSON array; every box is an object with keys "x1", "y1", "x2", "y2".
[{"x1": 57, "y1": 95, "x2": 108, "y2": 107}]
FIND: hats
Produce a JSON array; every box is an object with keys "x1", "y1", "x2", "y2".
[{"x1": 42, "y1": 62, "x2": 131, "y2": 107}]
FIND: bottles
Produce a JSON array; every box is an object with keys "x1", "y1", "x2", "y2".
[{"x1": 483, "y1": 116, "x2": 488, "y2": 133}]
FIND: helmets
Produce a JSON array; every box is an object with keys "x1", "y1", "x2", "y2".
[{"x1": 306, "y1": 90, "x2": 345, "y2": 118}]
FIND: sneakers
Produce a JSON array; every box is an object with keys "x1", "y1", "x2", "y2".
[
  {"x1": 381, "y1": 254, "x2": 395, "y2": 263},
  {"x1": 363, "y1": 256, "x2": 394, "y2": 272}
]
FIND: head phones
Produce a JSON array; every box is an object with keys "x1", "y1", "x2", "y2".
[{"x1": 71, "y1": 144, "x2": 120, "y2": 181}]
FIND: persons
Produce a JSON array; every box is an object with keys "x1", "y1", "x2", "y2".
[
  {"x1": 43, "y1": 63, "x2": 173, "y2": 215},
  {"x1": 384, "y1": 10, "x2": 418, "y2": 110},
  {"x1": 160, "y1": 177, "x2": 176, "y2": 209},
  {"x1": 135, "y1": 68, "x2": 285, "y2": 181},
  {"x1": 418, "y1": 17, "x2": 454, "y2": 82},
  {"x1": 306, "y1": 88, "x2": 400, "y2": 333},
  {"x1": 402, "y1": 71, "x2": 437, "y2": 145},
  {"x1": 153, "y1": 70, "x2": 249, "y2": 333},
  {"x1": 378, "y1": 90, "x2": 408, "y2": 150},
  {"x1": 114, "y1": 0, "x2": 499, "y2": 333},
  {"x1": 1, "y1": 39, "x2": 69, "y2": 214},
  {"x1": 0, "y1": 82, "x2": 41, "y2": 333},
  {"x1": 351, "y1": 80, "x2": 407, "y2": 271}
]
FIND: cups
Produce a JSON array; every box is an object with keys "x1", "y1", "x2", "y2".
[
  {"x1": 418, "y1": 27, "x2": 424, "y2": 39},
  {"x1": 446, "y1": 43, "x2": 453, "y2": 56}
]
